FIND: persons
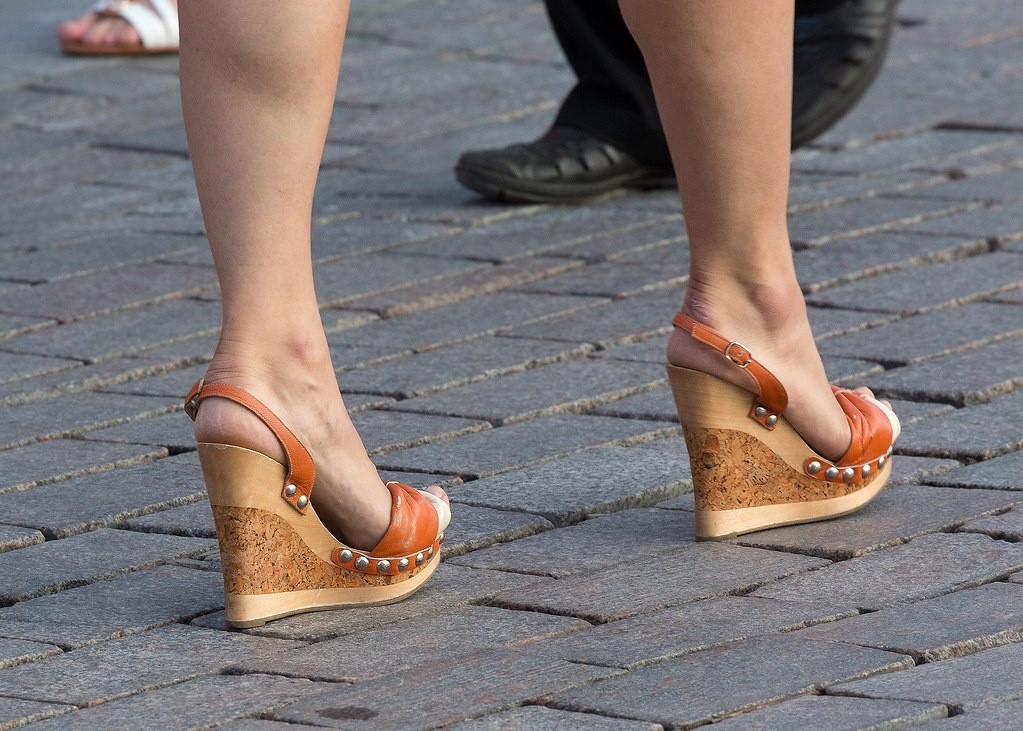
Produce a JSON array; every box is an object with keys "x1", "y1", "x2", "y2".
[
  {"x1": 177, "y1": 0, "x2": 901, "y2": 632},
  {"x1": 58, "y1": 0, "x2": 179, "y2": 53},
  {"x1": 454, "y1": 0, "x2": 896, "y2": 204}
]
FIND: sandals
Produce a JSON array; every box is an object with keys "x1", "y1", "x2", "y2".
[{"x1": 63, "y1": 0, "x2": 179, "y2": 54}]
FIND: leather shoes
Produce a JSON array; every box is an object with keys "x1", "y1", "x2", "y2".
[
  {"x1": 792, "y1": 0, "x2": 897, "y2": 150},
  {"x1": 456, "y1": 123, "x2": 678, "y2": 204}
]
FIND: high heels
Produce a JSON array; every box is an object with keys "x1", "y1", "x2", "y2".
[
  {"x1": 184, "y1": 378, "x2": 451, "y2": 627},
  {"x1": 666, "y1": 312, "x2": 901, "y2": 540}
]
